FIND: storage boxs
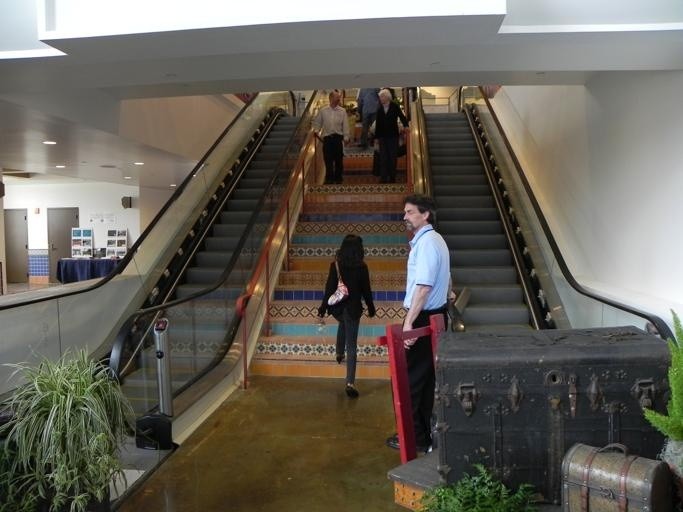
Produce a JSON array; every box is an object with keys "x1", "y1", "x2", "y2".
[
  {"x1": 559, "y1": 444, "x2": 670, "y2": 512},
  {"x1": 434, "y1": 326, "x2": 671, "y2": 512}
]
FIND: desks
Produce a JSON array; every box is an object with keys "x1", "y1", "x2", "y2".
[{"x1": 58, "y1": 258, "x2": 122, "y2": 285}]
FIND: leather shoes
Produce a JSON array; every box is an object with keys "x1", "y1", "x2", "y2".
[{"x1": 385, "y1": 438, "x2": 429, "y2": 451}]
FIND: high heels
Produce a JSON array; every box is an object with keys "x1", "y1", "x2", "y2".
[
  {"x1": 346, "y1": 386, "x2": 359, "y2": 398},
  {"x1": 337, "y1": 353, "x2": 345, "y2": 363}
]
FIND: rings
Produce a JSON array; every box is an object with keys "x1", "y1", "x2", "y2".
[{"x1": 407, "y1": 339, "x2": 410, "y2": 342}]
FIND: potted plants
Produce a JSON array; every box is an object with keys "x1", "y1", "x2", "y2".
[{"x1": 0, "y1": 345, "x2": 139, "y2": 512}]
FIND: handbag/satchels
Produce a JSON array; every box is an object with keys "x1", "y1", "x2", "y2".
[
  {"x1": 327, "y1": 284, "x2": 349, "y2": 306},
  {"x1": 397, "y1": 144, "x2": 407, "y2": 157}
]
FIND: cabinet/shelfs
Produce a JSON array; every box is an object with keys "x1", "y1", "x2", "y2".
[{"x1": 71, "y1": 228, "x2": 127, "y2": 258}]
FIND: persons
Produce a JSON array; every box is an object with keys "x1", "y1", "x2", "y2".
[
  {"x1": 314, "y1": 234, "x2": 377, "y2": 398},
  {"x1": 385, "y1": 194, "x2": 457, "y2": 453},
  {"x1": 313, "y1": 87, "x2": 410, "y2": 186}
]
